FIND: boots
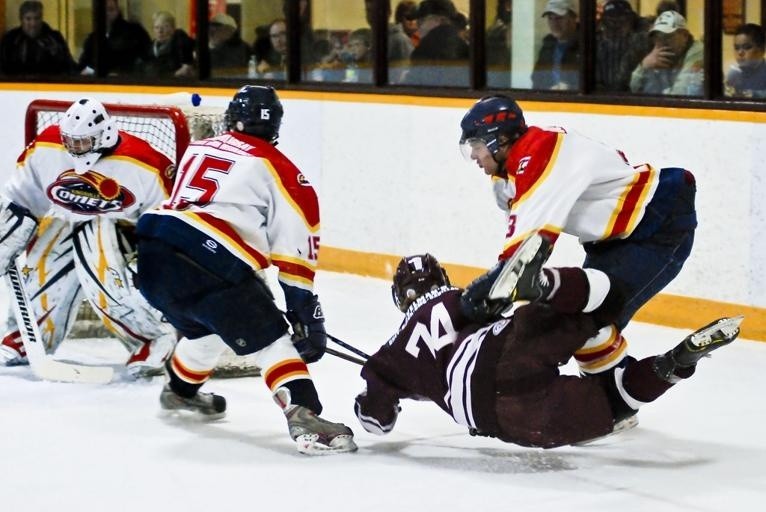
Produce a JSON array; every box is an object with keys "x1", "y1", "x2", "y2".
[
  {"x1": 159, "y1": 361, "x2": 225, "y2": 415},
  {"x1": 501, "y1": 235, "x2": 549, "y2": 303},
  {"x1": 651, "y1": 317, "x2": 740, "y2": 381},
  {"x1": 271, "y1": 387, "x2": 353, "y2": 446}
]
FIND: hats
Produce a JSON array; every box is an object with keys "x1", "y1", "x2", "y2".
[
  {"x1": 602, "y1": 1, "x2": 643, "y2": 31},
  {"x1": 208, "y1": 13, "x2": 236, "y2": 28},
  {"x1": 648, "y1": 10, "x2": 686, "y2": 33},
  {"x1": 541, "y1": 0, "x2": 576, "y2": 17},
  {"x1": 406, "y1": 0, "x2": 456, "y2": 20}
]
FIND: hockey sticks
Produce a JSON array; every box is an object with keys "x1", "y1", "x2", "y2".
[{"x1": 2, "y1": 262, "x2": 116, "y2": 387}]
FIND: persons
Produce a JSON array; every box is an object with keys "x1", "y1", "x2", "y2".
[
  {"x1": 353, "y1": 253, "x2": 744, "y2": 450},
  {"x1": 0, "y1": 1, "x2": 78, "y2": 79},
  {"x1": 459, "y1": 94, "x2": 695, "y2": 435},
  {"x1": 0, "y1": 101, "x2": 175, "y2": 380},
  {"x1": 78, "y1": 1, "x2": 766, "y2": 100},
  {"x1": 131, "y1": 85, "x2": 357, "y2": 455}
]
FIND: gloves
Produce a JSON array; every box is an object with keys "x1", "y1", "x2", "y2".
[
  {"x1": 285, "y1": 296, "x2": 325, "y2": 364},
  {"x1": 459, "y1": 261, "x2": 511, "y2": 322}
]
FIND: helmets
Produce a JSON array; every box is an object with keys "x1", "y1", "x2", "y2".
[
  {"x1": 58, "y1": 98, "x2": 117, "y2": 175},
  {"x1": 226, "y1": 84, "x2": 281, "y2": 140},
  {"x1": 391, "y1": 254, "x2": 450, "y2": 311},
  {"x1": 459, "y1": 95, "x2": 525, "y2": 154},
  {"x1": 395, "y1": 3, "x2": 417, "y2": 24}
]
